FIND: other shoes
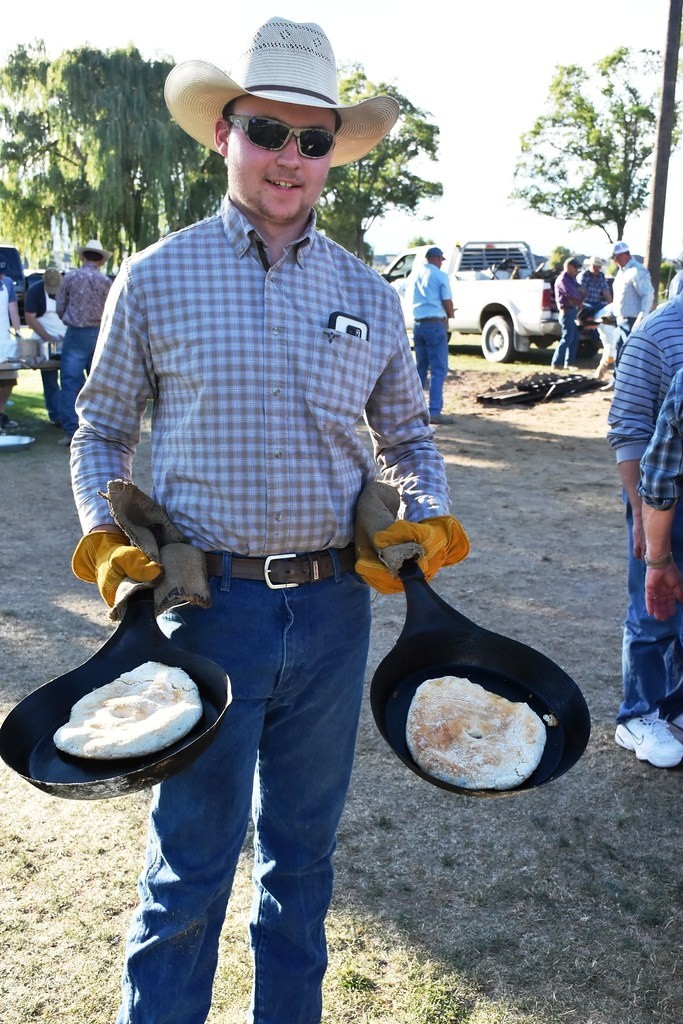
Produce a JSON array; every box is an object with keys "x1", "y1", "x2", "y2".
[
  {"x1": 0, "y1": 413, "x2": 20, "y2": 429},
  {"x1": 429, "y1": 413, "x2": 455, "y2": 425},
  {"x1": 599, "y1": 382, "x2": 615, "y2": 392},
  {"x1": 58, "y1": 435, "x2": 73, "y2": 447},
  {"x1": 50, "y1": 420, "x2": 63, "y2": 427}
]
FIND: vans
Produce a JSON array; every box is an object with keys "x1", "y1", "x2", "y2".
[{"x1": 0, "y1": 245, "x2": 30, "y2": 322}]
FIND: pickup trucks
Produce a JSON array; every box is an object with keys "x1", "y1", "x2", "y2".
[{"x1": 382, "y1": 241, "x2": 616, "y2": 362}]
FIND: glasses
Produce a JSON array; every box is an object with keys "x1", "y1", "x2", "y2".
[{"x1": 221, "y1": 110, "x2": 339, "y2": 159}]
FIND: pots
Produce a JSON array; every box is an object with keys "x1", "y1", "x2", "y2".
[
  {"x1": 1, "y1": 580, "x2": 232, "y2": 802},
  {"x1": 368, "y1": 557, "x2": 591, "y2": 797}
]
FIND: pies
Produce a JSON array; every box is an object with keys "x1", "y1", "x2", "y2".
[
  {"x1": 53, "y1": 661, "x2": 202, "y2": 759},
  {"x1": 407, "y1": 675, "x2": 547, "y2": 790}
]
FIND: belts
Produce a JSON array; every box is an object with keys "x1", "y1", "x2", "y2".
[{"x1": 187, "y1": 543, "x2": 360, "y2": 590}]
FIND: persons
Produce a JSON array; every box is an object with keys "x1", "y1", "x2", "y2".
[
  {"x1": 577, "y1": 302, "x2": 616, "y2": 381},
  {"x1": 413, "y1": 246, "x2": 455, "y2": 424},
  {"x1": 669, "y1": 262, "x2": 683, "y2": 301},
  {"x1": 606, "y1": 286, "x2": 683, "y2": 768},
  {"x1": 599, "y1": 242, "x2": 654, "y2": 390},
  {"x1": 56, "y1": 239, "x2": 113, "y2": 446},
  {"x1": 69, "y1": 19, "x2": 469, "y2": 1024},
  {"x1": 576, "y1": 256, "x2": 613, "y2": 311},
  {"x1": 24, "y1": 266, "x2": 61, "y2": 426},
  {"x1": 550, "y1": 256, "x2": 587, "y2": 372},
  {"x1": 0, "y1": 253, "x2": 20, "y2": 429}
]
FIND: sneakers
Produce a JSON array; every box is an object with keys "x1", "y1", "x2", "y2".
[
  {"x1": 614, "y1": 709, "x2": 683, "y2": 767},
  {"x1": 662, "y1": 711, "x2": 682, "y2": 732}
]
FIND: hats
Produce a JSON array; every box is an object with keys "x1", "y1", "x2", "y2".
[
  {"x1": 424, "y1": 247, "x2": 445, "y2": 261},
  {"x1": 564, "y1": 257, "x2": 583, "y2": 268},
  {"x1": 583, "y1": 256, "x2": 608, "y2": 266},
  {"x1": 76, "y1": 238, "x2": 114, "y2": 266},
  {"x1": 43, "y1": 266, "x2": 63, "y2": 298},
  {"x1": 609, "y1": 240, "x2": 632, "y2": 257},
  {"x1": 161, "y1": 15, "x2": 403, "y2": 171}
]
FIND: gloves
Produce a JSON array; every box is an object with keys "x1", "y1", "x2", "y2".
[
  {"x1": 69, "y1": 529, "x2": 167, "y2": 609},
  {"x1": 350, "y1": 512, "x2": 471, "y2": 597}
]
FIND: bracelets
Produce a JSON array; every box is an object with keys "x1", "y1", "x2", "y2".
[{"x1": 644, "y1": 551, "x2": 674, "y2": 568}]
